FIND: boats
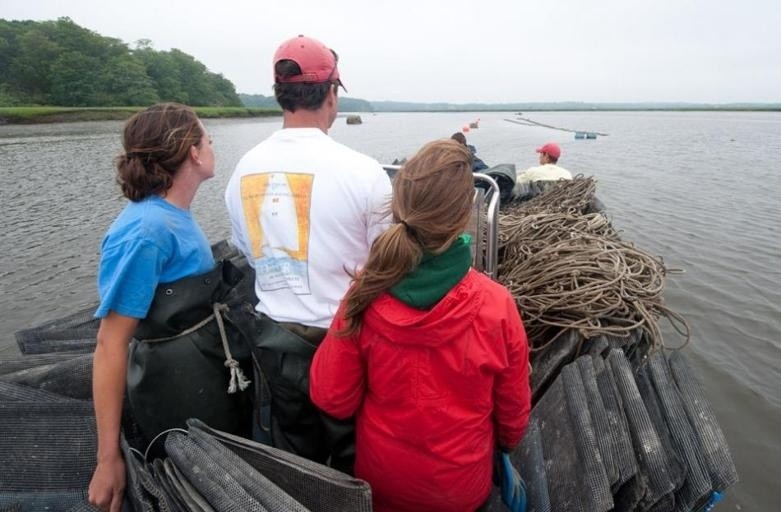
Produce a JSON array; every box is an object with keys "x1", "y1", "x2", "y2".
[
  {"x1": 346, "y1": 115, "x2": 361, "y2": 124},
  {"x1": 0, "y1": 165, "x2": 740, "y2": 511}
]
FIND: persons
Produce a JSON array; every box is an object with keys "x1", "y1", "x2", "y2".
[
  {"x1": 308, "y1": 139, "x2": 530, "y2": 512},
  {"x1": 449, "y1": 132, "x2": 489, "y2": 172},
  {"x1": 516, "y1": 139, "x2": 575, "y2": 186},
  {"x1": 229, "y1": 35, "x2": 399, "y2": 477},
  {"x1": 85, "y1": 99, "x2": 226, "y2": 511}
]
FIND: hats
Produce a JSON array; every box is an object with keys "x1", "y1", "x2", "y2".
[
  {"x1": 536, "y1": 143, "x2": 560, "y2": 158},
  {"x1": 273, "y1": 36, "x2": 349, "y2": 94}
]
